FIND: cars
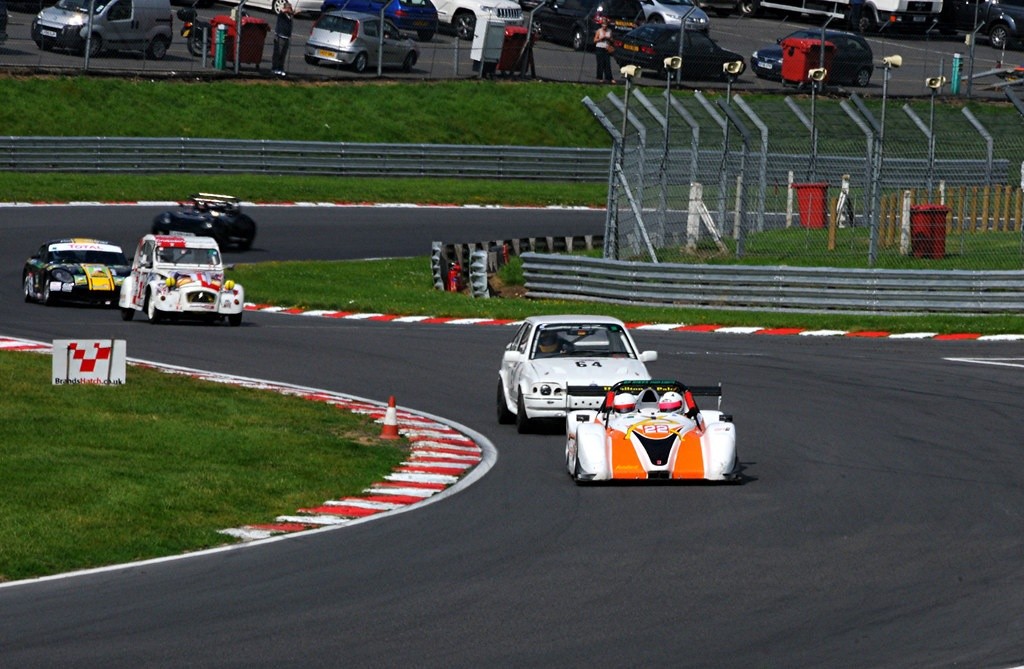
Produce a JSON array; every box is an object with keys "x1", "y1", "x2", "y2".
[
  {"x1": 639, "y1": 0, "x2": 711, "y2": 33},
  {"x1": 527, "y1": 0, "x2": 646, "y2": 52},
  {"x1": 401, "y1": 0, "x2": 526, "y2": 42},
  {"x1": 221, "y1": 0, "x2": 324, "y2": 21},
  {"x1": 750, "y1": 28, "x2": 875, "y2": 88},
  {"x1": 304, "y1": 10, "x2": 420, "y2": 74},
  {"x1": 320, "y1": 0, "x2": 439, "y2": 43},
  {"x1": 693, "y1": 0, "x2": 741, "y2": 19},
  {"x1": 496, "y1": 314, "x2": 659, "y2": 435},
  {"x1": 942, "y1": 0, "x2": 1024, "y2": 50},
  {"x1": 613, "y1": 24, "x2": 746, "y2": 82}
]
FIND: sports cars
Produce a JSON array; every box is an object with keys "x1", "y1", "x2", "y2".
[
  {"x1": 119, "y1": 233, "x2": 244, "y2": 328},
  {"x1": 21, "y1": 237, "x2": 134, "y2": 310},
  {"x1": 151, "y1": 192, "x2": 257, "y2": 254},
  {"x1": 564, "y1": 379, "x2": 738, "y2": 487}
]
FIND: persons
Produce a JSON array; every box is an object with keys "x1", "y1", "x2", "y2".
[
  {"x1": 539, "y1": 331, "x2": 558, "y2": 352},
  {"x1": 271, "y1": 3, "x2": 293, "y2": 76},
  {"x1": 594, "y1": 18, "x2": 616, "y2": 83},
  {"x1": 614, "y1": 393, "x2": 638, "y2": 417},
  {"x1": 659, "y1": 391, "x2": 685, "y2": 415}
]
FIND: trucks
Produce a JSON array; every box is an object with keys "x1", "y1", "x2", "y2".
[{"x1": 734, "y1": 0, "x2": 944, "y2": 41}]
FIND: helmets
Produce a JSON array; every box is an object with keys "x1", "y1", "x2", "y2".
[
  {"x1": 198, "y1": 202, "x2": 206, "y2": 209},
  {"x1": 658, "y1": 392, "x2": 685, "y2": 416},
  {"x1": 613, "y1": 393, "x2": 638, "y2": 413},
  {"x1": 539, "y1": 333, "x2": 558, "y2": 353}
]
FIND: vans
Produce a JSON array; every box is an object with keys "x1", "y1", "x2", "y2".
[{"x1": 30, "y1": 0, "x2": 173, "y2": 61}]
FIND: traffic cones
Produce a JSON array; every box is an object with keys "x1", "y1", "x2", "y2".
[{"x1": 379, "y1": 396, "x2": 402, "y2": 440}]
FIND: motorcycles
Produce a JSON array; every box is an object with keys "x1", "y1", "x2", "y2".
[{"x1": 177, "y1": 7, "x2": 250, "y2": 57}]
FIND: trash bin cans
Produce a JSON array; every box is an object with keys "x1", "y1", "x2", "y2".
[
  {"x1": 781, "y1": 38, "x2": 837, "y2": 90},
  {"x1": 909, "y1": 204, "x2": 951, "y2": 260},
  {"x1": 496, "y1": 26, "x2": 536, "y2": 77},
  {"x1": 209, "y1": 14, "x2": 270, "y2": 70},
  {"x1": 791, "y1": 183, "x2": 831, "y2": 229}
]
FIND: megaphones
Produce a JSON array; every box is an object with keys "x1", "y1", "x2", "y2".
[
  {"x1": 723, "y1": 61, "x2": 744, "y2": 74},
  {"x1": 665, "y1": 57, "x2": 681, "y2": 68},
  {"x1": 926, "y1": 76, "x2": 946, "y2": 88},
  {"x1": 621, "y1": 65, "x2": 642, "y2": 78},
  {"x1": 883, "y1": 54, "x2": 902, "y2": 67},
  {"x1": 809, "y1": 68, "x2": 827, "y2": 80}
]
